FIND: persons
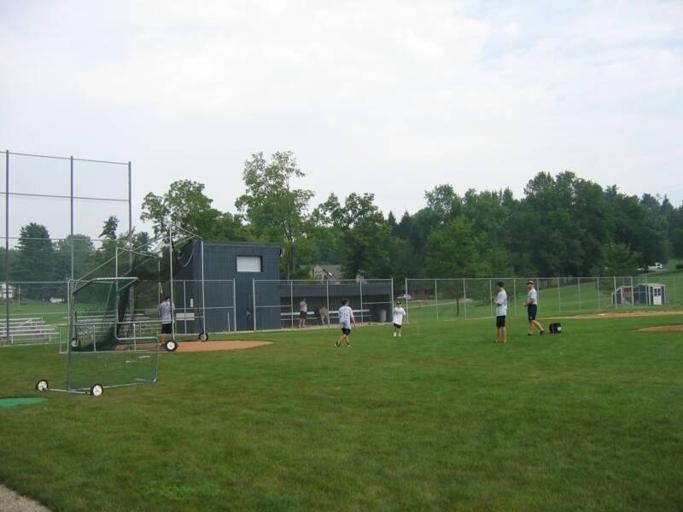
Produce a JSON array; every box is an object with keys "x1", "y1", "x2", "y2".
[
  {"x1": 158, "y1": 296, "x2": 175, "y2": 336},
  {"x1": 319, "y1": 303, "x2": 330, "y2": 325},
  {"x1": 246, "y1": 306, "x2": 252, "y2": 328},
  {"x1": 298, "y1": 296, "x2": 307, "y2": 328},
  {"x1": 334, "y1": 297, "x2": 356, "y2": 348},
  {"x1": 493, "y1": 281, "x2": 508, "y2": 344},
  {"x1": 392, "y1": 301, "x2": 406, "y2": 337},
  {"x1": 315, "y1": 303, "x2": 323, "y2": 326},
  {"x1": 523, "y1": 279, "x2": 546, "y2": 337}
]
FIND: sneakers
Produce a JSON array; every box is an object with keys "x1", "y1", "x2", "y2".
[
  {"x1": 540, "y1": 329, "x2": 544, "y2": 336},
  {"x1": 334, "y1": 341, "x2": 352, "y2": 349}
]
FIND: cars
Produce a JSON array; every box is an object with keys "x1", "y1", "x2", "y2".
[
  {"x1": 637, "y1": 262, "x2": 662, "y2": 272},
  {"x1": 397, "y1": 294, "x2": 412, "y2": 301}
]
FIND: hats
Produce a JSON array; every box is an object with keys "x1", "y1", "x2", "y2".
[{"x1": 525, "y1": 279, "x2": 535, "y2": 285}]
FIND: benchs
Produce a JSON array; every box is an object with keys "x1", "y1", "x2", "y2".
[
  {"x1": 280, "y1": 309, "x2": 376, "y2": 328},
  {"x1": 0, "y1": 313, "x2": 163, "y2": 345}
]
